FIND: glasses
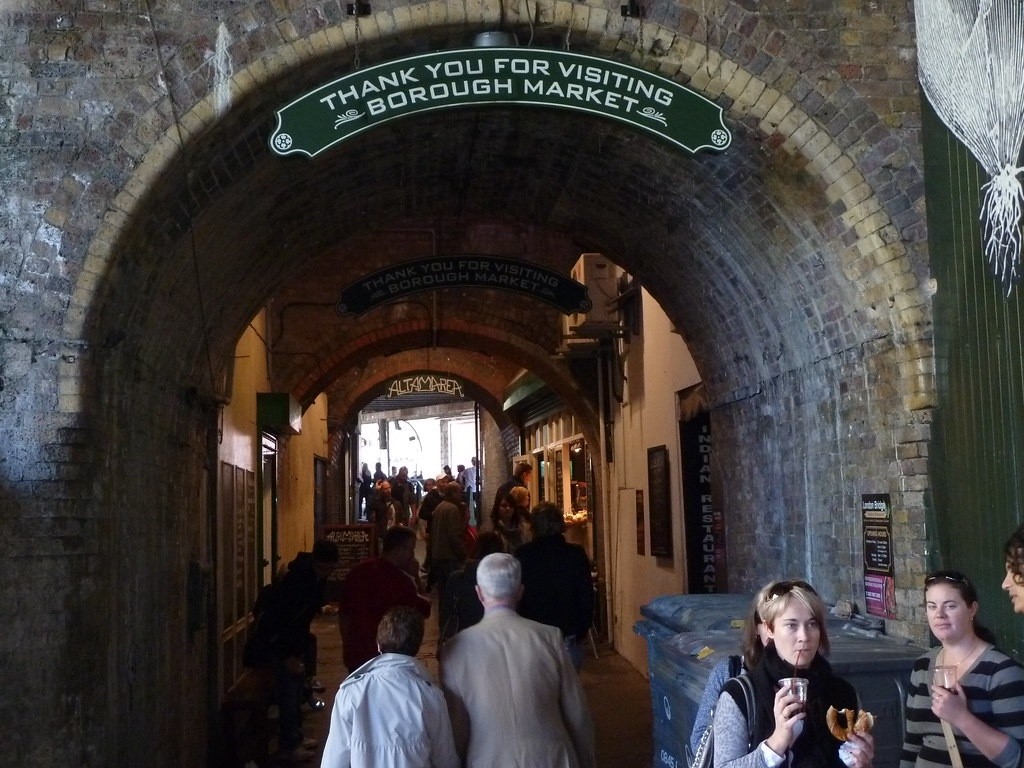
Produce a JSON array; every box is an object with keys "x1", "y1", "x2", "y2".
[
  {"x1": 765, "y1": 580, "x2": 818, "y2": 606},
  {"x1": 924, "y1": 572, "x2": 969, "y2": 591}
]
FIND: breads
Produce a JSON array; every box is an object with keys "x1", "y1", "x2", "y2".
[{"x1": 826, "y1": 705, "x2": 874, "y2": 741}]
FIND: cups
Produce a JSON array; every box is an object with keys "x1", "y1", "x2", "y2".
[
  {"x1": 778, "y1": 677, "x2": 809, "y2": 720},
  {"x1": 931, "y1": 665, "x2": 957, "y2": 695}
]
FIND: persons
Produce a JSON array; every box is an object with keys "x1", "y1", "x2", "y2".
[
  {"x1": 319, "y1": 605, "x2": 461, "y2": 768},
  {"x1": 898, "y1": 520, "x2": 1024, "y2": 768},
  {"x1": 359, "y1": 457, "x2": 533, "y2": 646},
  {"x1": 337, "y1": 525, "x2": 433, "y2": 675},
  {"x1": 690, "y1": 578, "x2": 875, "y2": 768},
  {"x1": 513, "y1": 502, "x2": 594, "y2": 674},
  {"x1": 218, "y1": 540, "x2": 345, "y2": 768},
  {"x1": 436, "y1": 553, "x2": 596, "y2": 768}
]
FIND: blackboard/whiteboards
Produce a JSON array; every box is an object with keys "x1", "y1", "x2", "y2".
[{"x1": 321, "y1": 524, "x2": 377, "y2": 606}]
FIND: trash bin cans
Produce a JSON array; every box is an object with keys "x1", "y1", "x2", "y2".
[
  {"x1": 634, "y1": 594, "x2": 780, "y2": 767},
  {"x1": 663, "y1": 630, "x2": 933, "y2": 768}
]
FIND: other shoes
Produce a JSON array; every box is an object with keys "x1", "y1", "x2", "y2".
[
  {"x1": 299, "y1": 736, "x2": 320, "y2": 747},
  {"x1": 307, "y1": 693, "x2": 326, "y2": 711},
  {"x1": 305, "y1": 675, "x2": 325, "y2": 692},
  {"x1": 277, "y1": 744, "x2": 315, "y2": 761}
]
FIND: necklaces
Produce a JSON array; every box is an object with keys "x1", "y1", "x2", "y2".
[{"x1": 942, "y1": 636, "x2": 977, "y2": 666}]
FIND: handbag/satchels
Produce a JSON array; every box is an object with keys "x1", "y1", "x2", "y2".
[{"x1": 435, "y1": 570, "x2": 465, "y2": 662}]
